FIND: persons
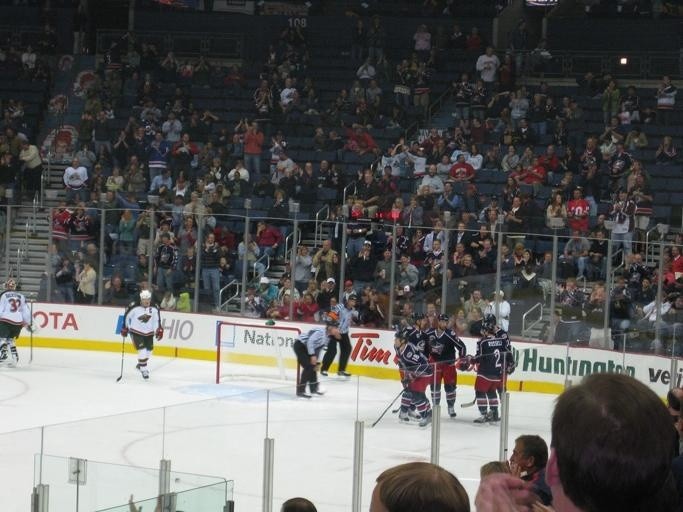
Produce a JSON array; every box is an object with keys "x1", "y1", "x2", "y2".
[
  {"x1": 1, "y1": 276, "x2": 33, "y2": 367},
  {"x1": 508, "y1": 433, "x2": 553, "y2": 506},
  {"x1": 1, "y1": 0, "x2": 683, "y2": 361},
  {"x1": 120, "y1": 287, "x2": 166, "y2": 380},
  {"x1": 668, "y1": 385, "x2": 683, "y2": 422},
  {"x1": 279, "y1": 496, "x2": 317, "y2": 512},
  {"x1": 367, "y1": 460, "x2": 471, "y2": 511},
  {"x1": 293, "y1": 321, "x2": 341, "y2": 399},
  {"x1": 472, "y1": 372, "x2": 651, "y2": 510},
  {"x1": 391, "y1": 311, "x2": 518, "y2": 429},
  {"x1": 319, "y1": 294, "x2": 360, "y2": 379},
  {"x1": 479, "y1": 457, "x2": 512, "y2": 475}
]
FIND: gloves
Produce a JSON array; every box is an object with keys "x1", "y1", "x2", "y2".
[
  {"x1": 121, "y1": 327, "x2": 128, "y2": 336},
  {"x1": 156, "y1": 328, "x2": 163, "y2": 341}
]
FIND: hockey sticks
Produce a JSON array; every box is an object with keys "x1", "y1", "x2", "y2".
[
  {"x1": 363, "y1": 387, "x2": 407, "y2": 428},
  {"x1": 117, "y1": 336, "x2": 124, "y2": 381},
  {"x1": 461, "y1": 397, "x2": 476, "y2": 407},
  {"x1": 28, "y1": 299, "x2": 32, "y2": 364}
]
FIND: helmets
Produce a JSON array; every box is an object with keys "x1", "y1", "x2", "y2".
[
  {"x1": 326, "y1": 294, "x2": 496, "y2": 340},
  {"x1": 4, "y1": 279, "x2": 16, "y2": 290},
  {"x1": 139, "y1": 290, "x2": 152, "y2": 299}
]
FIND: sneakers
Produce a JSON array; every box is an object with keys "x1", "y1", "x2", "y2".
[
  {"x1": 139, "y1": 366, "x2": 149, "y2": 378},
  {"x1": 11, "y1": 346, "x2": 18, "y2": 361},
  {"x1": 297, "y1": 370, "x2": 499, "y2": 427},
  {"x1": 0, "y1": 344, "x2": 8, "y2": 359}
]
